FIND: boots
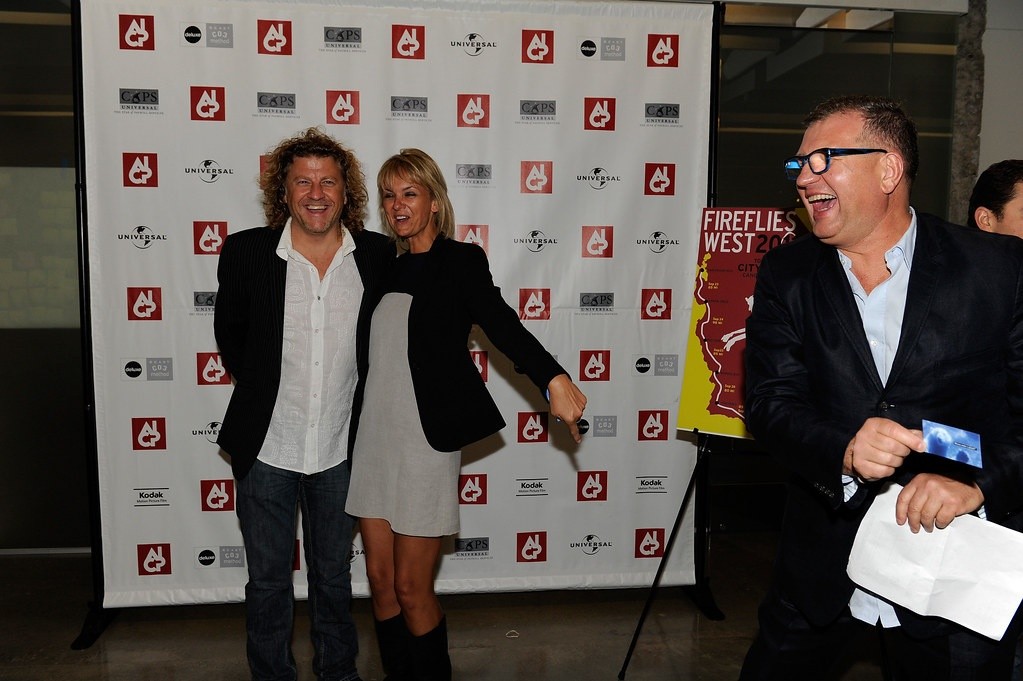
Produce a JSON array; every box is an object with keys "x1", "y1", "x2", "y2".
[{"x1": 373, "y1": 608, "x2": 451, "y2": 681}]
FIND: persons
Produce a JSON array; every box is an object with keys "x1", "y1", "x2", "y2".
[
  {"x1": 214, "y1": 127, "x2": 399, "y2": 681},
  {"x1": 966, "y1": 159, "x2": 1023, "y2": 239},
  {"x1": 740, "y1": 98, "x2": 1023, "y2": 681},
  {"x1": 342, "y1": 148, "x2": 588, "y2": 681}
]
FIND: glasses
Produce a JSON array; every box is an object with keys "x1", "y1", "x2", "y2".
[{"x1": 784, "y1": 148, "x2": 888, "y2": 181}]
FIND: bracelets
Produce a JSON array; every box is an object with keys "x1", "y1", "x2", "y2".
[{"x1": 851, "y1": 452, "x2": 877, "y2": 488}]
